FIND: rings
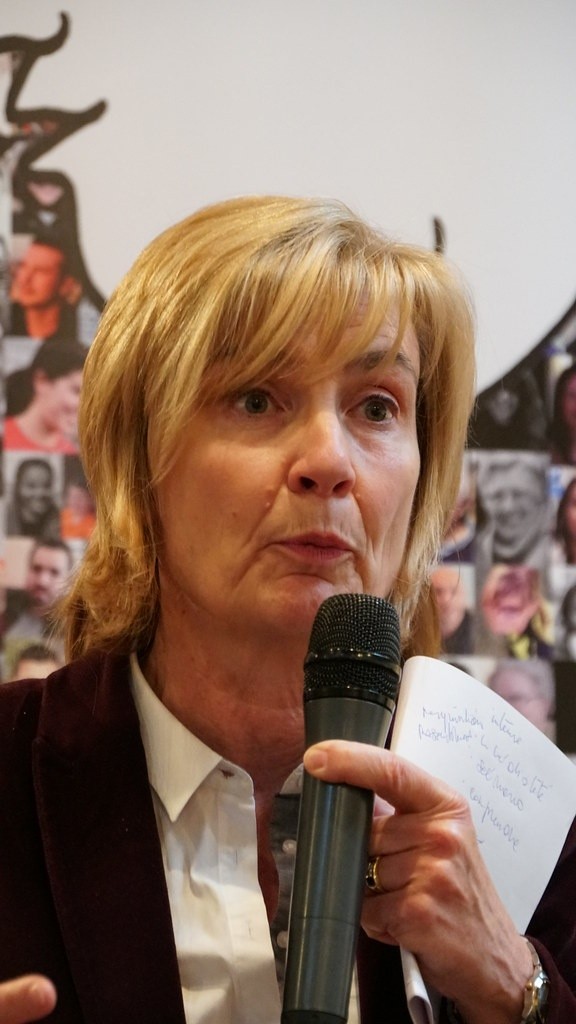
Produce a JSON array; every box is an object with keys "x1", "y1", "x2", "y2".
[{"x1": 365, "y1": 856, "x2": 387, "y2": 893}]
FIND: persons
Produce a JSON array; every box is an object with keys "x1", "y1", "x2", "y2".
[
  {"x1": 0, "y1": 196, "x2": 576, "y2": 1024},
  {"x1": 431, "y1": 364, "x2": 576, "y2": 736},
  {"x1": 0, "y1": 232, "x2": 89, "y2": 680}
]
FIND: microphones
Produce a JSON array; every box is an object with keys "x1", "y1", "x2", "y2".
[{"x1": 278, "y1": 590, "x2": 403, "y2": 1024}]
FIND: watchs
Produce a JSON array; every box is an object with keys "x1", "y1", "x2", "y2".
[{"x1": 520, "y1": 936, "x2": 550, "y2": 1024}]
